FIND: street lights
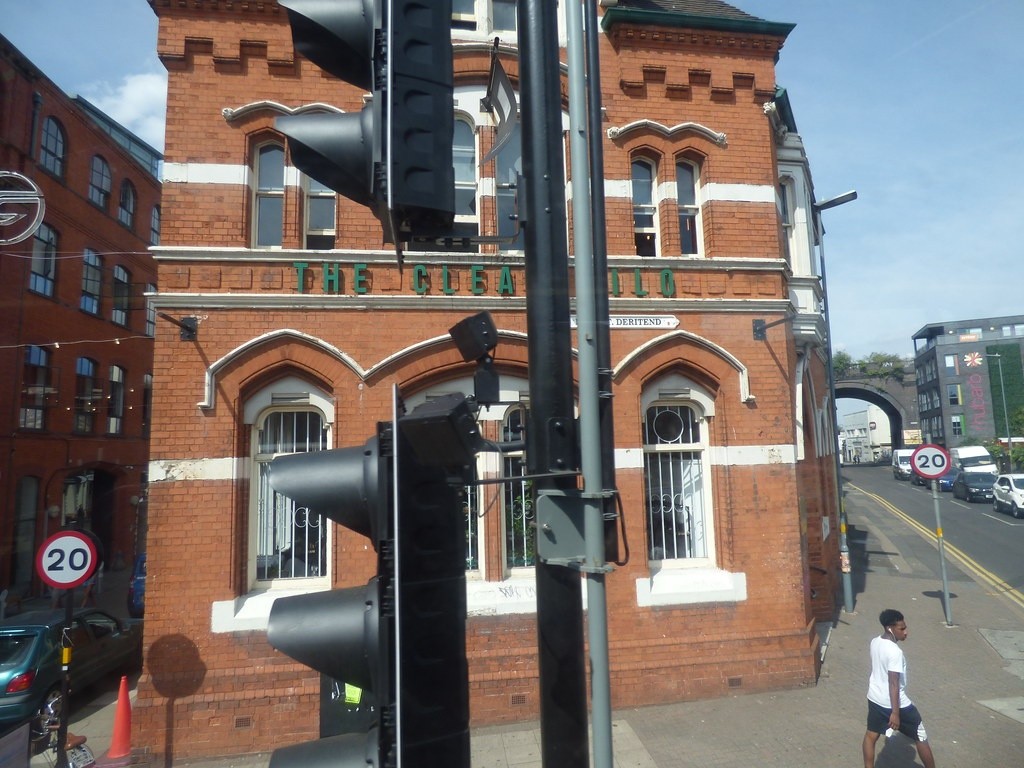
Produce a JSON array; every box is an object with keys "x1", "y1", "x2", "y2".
[{"x1": 809, "y1": 189, "x2": 862, "y2": 614}]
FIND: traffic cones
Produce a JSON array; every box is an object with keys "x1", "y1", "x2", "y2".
[{"x1": 107, "y1": 676, "x2": 132, "y2": 757}]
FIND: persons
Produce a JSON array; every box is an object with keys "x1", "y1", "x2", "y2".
[
  {"x1": 857, "y1": 455, "x2": 860, "y2": 464},
  {"x1": 862, "y1": 609, "x2": 936, "y2": 768},
  {"x1": 853, "y1": 455, "x2": 857, "y2": 464}
]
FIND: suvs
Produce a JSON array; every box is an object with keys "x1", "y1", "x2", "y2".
[{"x1": 993, "y1": 474, "x2": 1024, "y2": 518}]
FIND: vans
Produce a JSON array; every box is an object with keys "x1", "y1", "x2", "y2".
[
  {"x1": 949, "y1": 446, "x2": 999, "y2": 477},
  {"x1": 891, "y1": 449, "x2": 916, "y2": 481}
]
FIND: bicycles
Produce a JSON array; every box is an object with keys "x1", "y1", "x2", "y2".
[{"x1": 30, "y1": 692, "x2": 96, "y2": 768}]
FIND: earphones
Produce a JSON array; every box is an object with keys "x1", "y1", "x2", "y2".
[{"x1": 889, "y1": 628, "x2": 893, "y2": 633}]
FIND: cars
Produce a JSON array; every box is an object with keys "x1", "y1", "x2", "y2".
[
  {"x1": 952, "y1": 472, "x2": 995, "y2": 503},
  {"x1": 910, "y1": 466, "x2": 961, "y2": 492},
  {"x1": 0, "y1": 607, "x2": 144, "y2": 722},
  {"x1": 128, "y1": 553, "x2": 146, "y2": 618}
]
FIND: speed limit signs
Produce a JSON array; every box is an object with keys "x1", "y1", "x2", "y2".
[
  {"x1": 910, "y1": 443, "x2": 951, "y2": 479},
  {"x1": 31, "y1": 530, "x2": 98, "y2": 591}
]
